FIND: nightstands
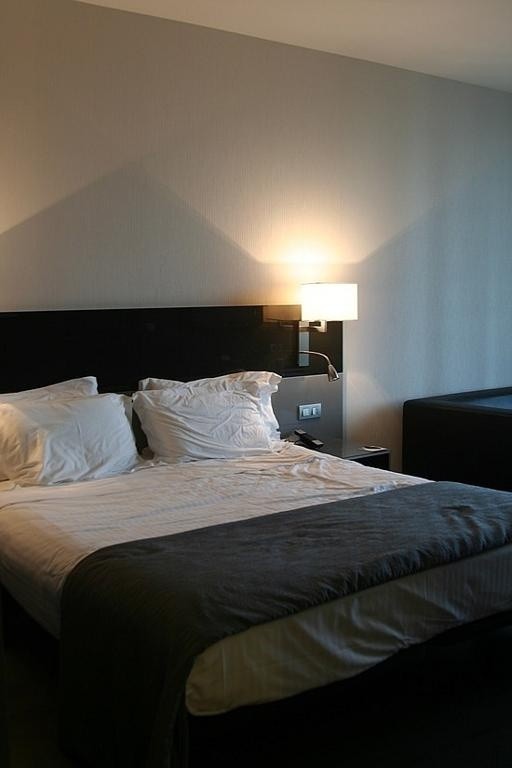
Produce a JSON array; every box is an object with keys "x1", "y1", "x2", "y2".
[{"x1": 292, "y1": 437, "x2": 390, "y2": 472}]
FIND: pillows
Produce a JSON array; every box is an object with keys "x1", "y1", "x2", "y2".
[{"x1": 1, "y1": 370, "x2": 283, "y2": 489}]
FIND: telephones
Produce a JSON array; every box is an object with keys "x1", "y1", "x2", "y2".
[{"x1": 294, "y1": 428, "x2": 325, "y2": 448}]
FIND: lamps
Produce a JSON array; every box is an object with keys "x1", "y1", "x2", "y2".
[{"x1": 297, "y1": 281, "x2": 358, "y2": 381}]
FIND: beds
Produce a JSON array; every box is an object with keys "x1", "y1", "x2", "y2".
[{"x1": 1, "y1": 304, "x2": 512, "y2": 767}]
